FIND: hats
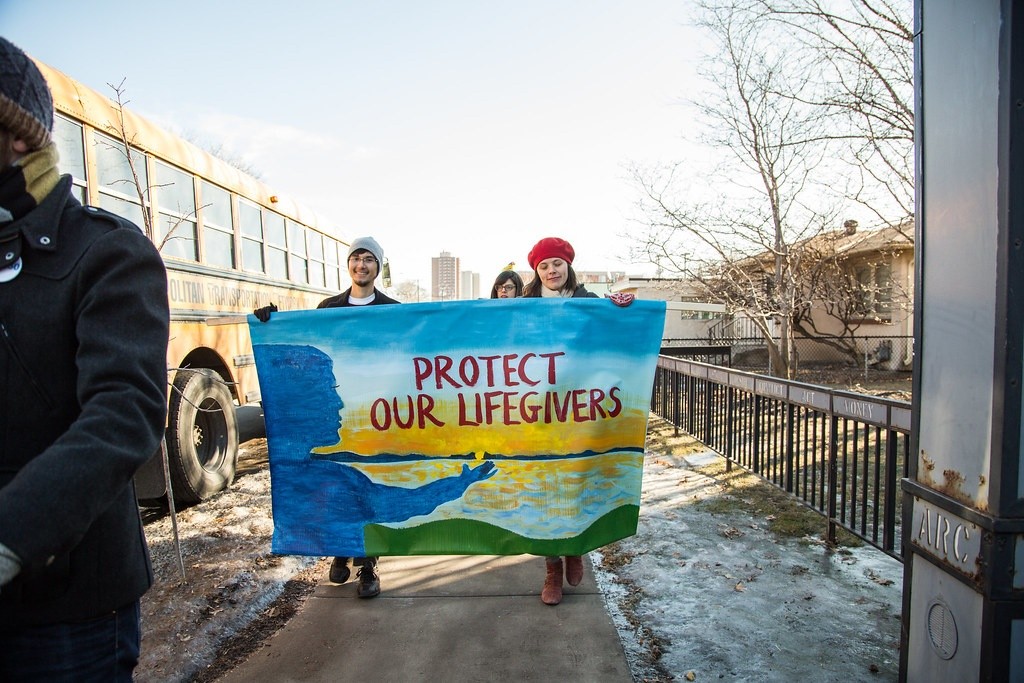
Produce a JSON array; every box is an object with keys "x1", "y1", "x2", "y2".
[
  {"x1": 0, "y1": 36, "x2": 54, "y2": 153},
  {"x1": 528, "y1": 237, "x2": 575, "y2": 272},
  {"x1": 347, "y1": 237, "x2": 384, "y2": 276}
]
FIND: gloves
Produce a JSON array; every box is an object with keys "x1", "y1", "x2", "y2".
[
  {"x1": 604, "y1": 292, "x2": 634, "y2": 306},
  {"x1": 255, "y1": 305, "x2": 277, "y2": 323}
]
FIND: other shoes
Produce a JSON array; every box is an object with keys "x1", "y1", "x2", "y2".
[
  {"x1": 565, "y1": 556, "x2": 584, "y2": 586},
  {"x1": 541, "y1": 560, "x2": 563, "y2": 604},
  {"x1": 330, "y1": 556, "x2": 352, "y2": 583},
  {"x1": 354, "y1": 565, "x2": 381, "y2": 598}
]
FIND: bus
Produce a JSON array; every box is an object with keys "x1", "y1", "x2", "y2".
[{"x1": 21, "y1": 53, "x2": 393, "y2": 504}]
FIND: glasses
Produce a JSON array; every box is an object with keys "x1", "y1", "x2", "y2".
[
  {"x1": 494, "y1": 285, "x2": 516, "y2": 292},
  {"x1": 348, "y1": 257, "x2": 377, "y2": 264}
]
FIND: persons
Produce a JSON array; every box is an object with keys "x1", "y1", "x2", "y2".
[
  {"x1": 490, "y1": 270, "x2": 524, "y2": 299},
  {"x1": 0, "y1": 39, "x2": 171, "y2": 682},
  {"x1": 518, "y1": 237, "x2": 636, "y2": 605},
  {"x1": 253, "y1": 236, "x2": 401, "y2": 599}
]
